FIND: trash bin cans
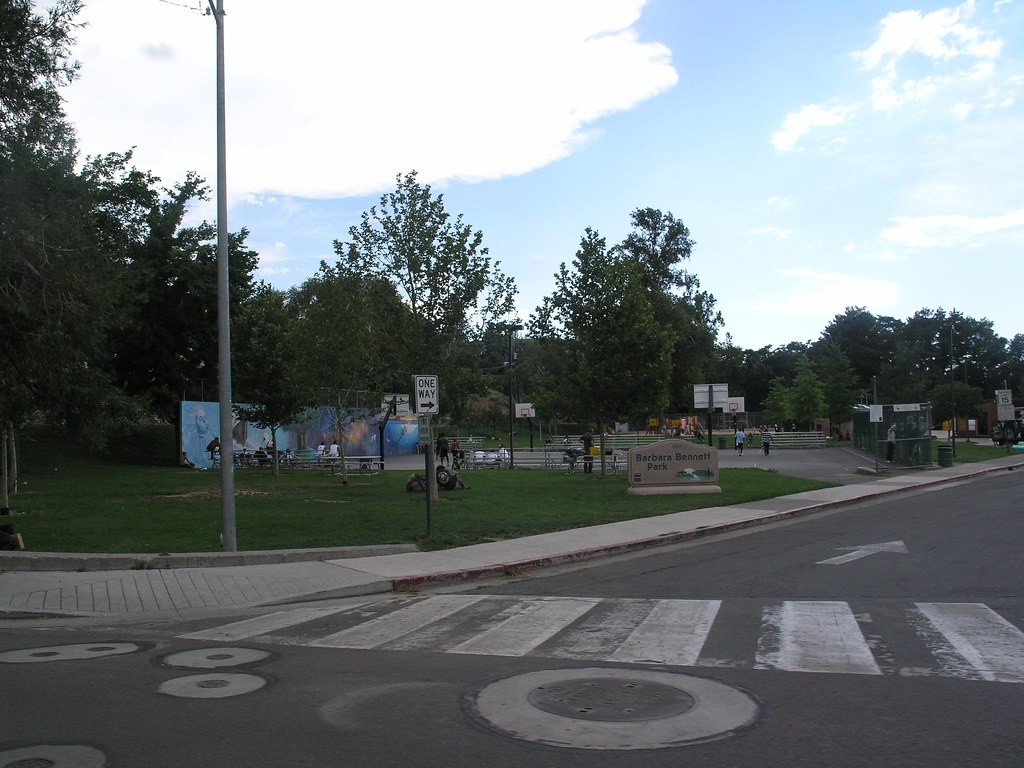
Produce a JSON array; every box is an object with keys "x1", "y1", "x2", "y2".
[
  {"x1": 938, "y1": 445, "x2": 953, "y2": 467},
  {"x1": 719, "y1": 438, "x2": 726, "y2": 450}
]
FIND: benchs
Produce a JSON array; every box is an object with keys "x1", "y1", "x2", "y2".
[
  {"x1": 544, "y1": 435, "x2": 698, "y2": 452},
  {"x1": 461, "y1": 452, "x2": 511, "y2": 469},
  {"x1": 433, "y1": 436, "x2": 486, "y2": 454},
  {"x1": 558, "y1": 461, "x2": 628, "y2": 476},
  {"x1": 204, "y1": 448, "x2": 386, "y2": 479},
  {"x1": 761, "y1": 431, "x2": 827, "y2": 449}
]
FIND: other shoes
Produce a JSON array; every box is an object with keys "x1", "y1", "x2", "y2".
[
  {"x1": 885, "y1": 460, "x2": 895, "y2": 463},
  {"x1": 739, "y1": 453, "x2": 743, "y2": 456},
  {"x1": 765, "y1": 453, "x2": 769, "y2": 456}
]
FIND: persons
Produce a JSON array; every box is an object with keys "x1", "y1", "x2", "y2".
[
  {"x1": 759, "y1": 423, "x2": 796, "y2": 432},
  {"x1": 886, "y1": 422, "x2": 897, "y2": 463},
  {"x1": 233, "y1": 441, "x2": 293, "y2": 469},
  {"x1": 436, "y1": 464, "x2": 471, "y2": 491},
  {"x1": 546, "y1": 433, "x2": 571, "y2": 444},
  {"x1": 436, "y1": 433, "x2": 509, "y2": 470},
  {"x1": 179, "y1": 452, "x2": 192, "y2": 467},
  {"x1": 762, "y1": 429, "x2": 774, "y2": 456},
  {"x1": 815, "y1": 420, "x2": 850, "y2": 441},
  {"x1": 663, "y1": 417, "x2": 704, "y2": 440},
  {"x1": 578, "y1": 429, "x2": 595, "y2": 473},
  {"x1": 318, "y1": 441, "x2": 340, "y2": 462},
  {"x1": 734, "y1": 428, "x2": 745, "y2": 456},
  {"x1": 207, "y1": 437, "x2": 220, "y2": 459}
]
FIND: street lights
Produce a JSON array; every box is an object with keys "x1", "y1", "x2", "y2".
[
  {"x1": 949, "y1": 324, "x2": 958, "y2": 458},
  {"x1": 495, "y1": 325, "x2": 525, "y2": 470}
]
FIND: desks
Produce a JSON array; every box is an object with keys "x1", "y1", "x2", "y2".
[{"x1": 321, "y1": 456, "x2": 381, "y2": 476}]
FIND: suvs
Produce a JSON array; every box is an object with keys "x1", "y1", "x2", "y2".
[{"x1": 991, "y1": 407, "x2": 1024, "y2": 446}]
